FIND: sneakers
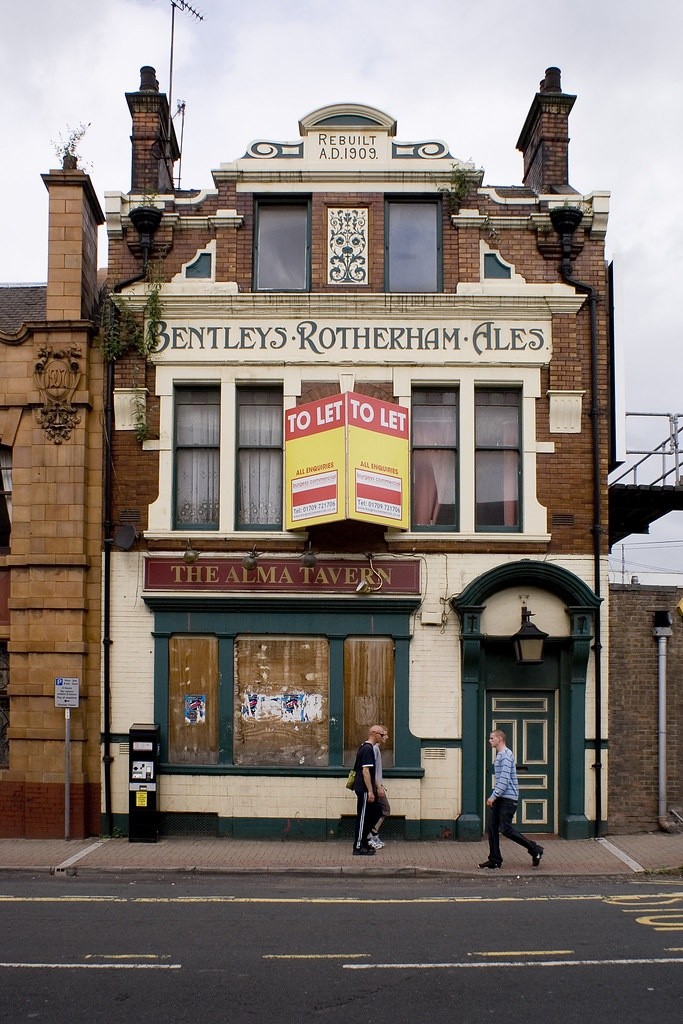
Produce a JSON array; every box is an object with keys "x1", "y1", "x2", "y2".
[
  {"x1": 532, "y1": 845, "x2": 545, "y2": 866},
  {"x1": 368, "y1": 837, "x2": 382, "y2": 850},
  {"x1": 374, "y1": 834, "x2": 385, "y2": 846},
  {"x1": 477, "y1": 860, "x2": 503, "y2": 870},
  {"x1": 353, "y1": 838, "x2": 376, "y2": 856}
]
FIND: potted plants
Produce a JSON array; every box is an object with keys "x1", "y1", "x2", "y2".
[
  {"x1": 436, "y1": 161, "x2": 479, "y2": 216},
  {"x1": 549, "y1": 199, "x2": 592, "y2": 233},
  {"x1": 129, "y1": 363, "x2": 160, "y2": 441},
  {"x1": 127, "y1": 181, "x2": 163, "y2": 233},
  {"x1": 50, "y1": 122, "x2": 94, "y2": 169}
]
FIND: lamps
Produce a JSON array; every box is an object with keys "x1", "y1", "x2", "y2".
[
  {"x1": 182, "y1": 537, "x2": 200, "y2": 564},
  {"x1": 356, "y1": 554, "x2": 383, "y2": 594},
  {"x1": 115, "y1": 525, "x2": 136, "y2": 552},
  {"x1": 241, "y1": 544, "x2": 263, "y2": 570},
  {"x1": 299, "y1": 539, "x2": 318, "y2": 570},
  {"x1": 510, "y1": 607, "x2": 549, "y2": 666}
]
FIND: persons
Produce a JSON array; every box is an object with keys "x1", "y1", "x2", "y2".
[
  {"x1": 478, "y1": 730, "x2": 544, "y2": 870},
  {"x1": 353, "y1": 725, "x2": 391, "y2": 857}
]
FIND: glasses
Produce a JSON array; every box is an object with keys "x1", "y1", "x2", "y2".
[{"x1": 376, "y1": 733, "x2": 384, "y2": 737}]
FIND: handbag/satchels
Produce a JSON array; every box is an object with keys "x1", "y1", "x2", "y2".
[{"x1": 345, "y1": 769, "x2": 356, "y2": 791}]
